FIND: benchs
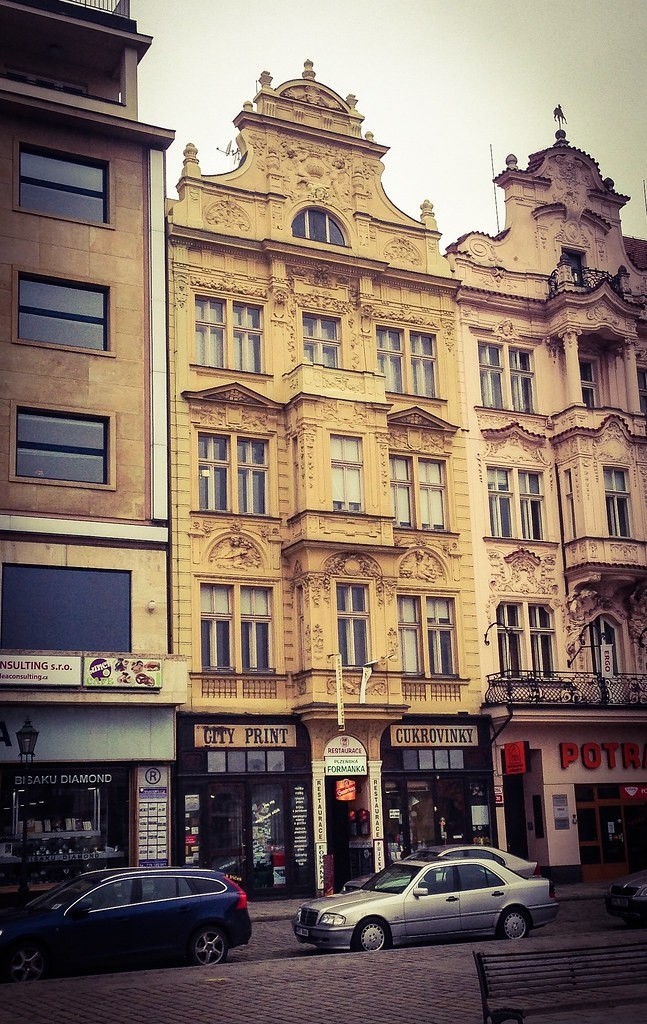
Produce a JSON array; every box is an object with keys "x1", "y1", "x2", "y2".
[{"x1": 472, "y1": 941, "x2": 647, "y2": 1024}]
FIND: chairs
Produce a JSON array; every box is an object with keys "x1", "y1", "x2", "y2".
[
  {"x1": 119, "y1": 882, "x2": 132, "y2": 905},
  {"x1": 419, "y1": 872, "x2": 438, "y2": 890},
  {"x1": 442, "y1": 871, "x2": 456, "y2": 890}
]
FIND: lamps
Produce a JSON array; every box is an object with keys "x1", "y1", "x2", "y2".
[{"x1": 147, "y1": 600, "x2": 156, "y2": 613}]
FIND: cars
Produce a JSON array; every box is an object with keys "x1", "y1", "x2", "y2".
[
  {"x1": 209, "y1": 847, "x2": 248, "y2": 877},
  {"x1": 605, "y1": 871, "x2": 647, "y2": 921},
  {"x1": 340, "y1": 845, "x2": 537, "y2": 896},
  {"x1": 291, "y1": 857, "x2": 559, "y2": 954},
  {"x1": 1, "y1": 863, "x2": 253, "y2": 981}
]
